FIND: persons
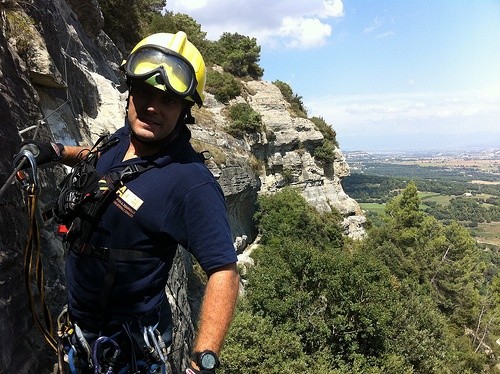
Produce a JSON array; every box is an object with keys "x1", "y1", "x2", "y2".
[{"x1": 14, "y1": 28, "x2": 241, "y2": 374}]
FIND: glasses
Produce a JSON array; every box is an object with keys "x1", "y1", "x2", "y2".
[{"x1": 127, "y1": 45, "x2": 203, "y2": 109}]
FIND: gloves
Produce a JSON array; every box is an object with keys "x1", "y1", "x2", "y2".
[{"x1": 12, "y1": 140, "x2": 65, "y2": 168}]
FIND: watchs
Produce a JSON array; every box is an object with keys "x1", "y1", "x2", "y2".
[{"x1": 189, "y1": 349, "x2": 221, "y2": 374}]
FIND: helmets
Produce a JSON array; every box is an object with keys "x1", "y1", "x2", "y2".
[{"x1": 127, "y1": 30, "x2": 205, "y2": 104}]
